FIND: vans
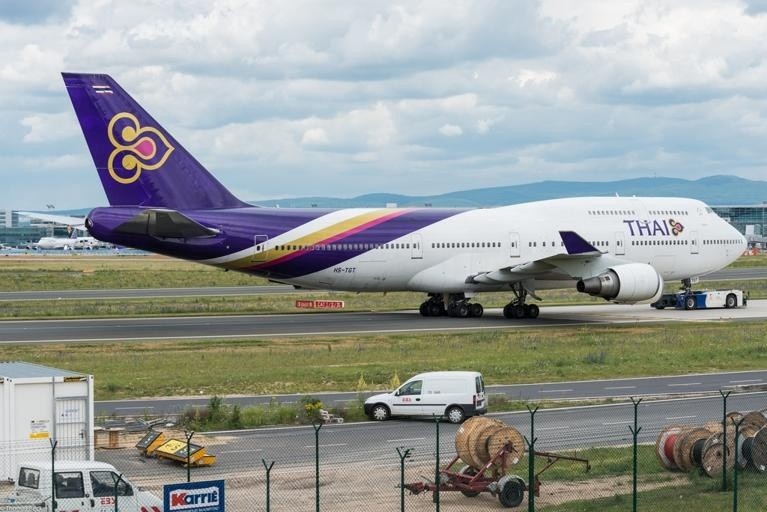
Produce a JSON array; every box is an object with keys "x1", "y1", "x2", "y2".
[{"x1": 363, "y1": 370, "x2": 488, "y2": 424}]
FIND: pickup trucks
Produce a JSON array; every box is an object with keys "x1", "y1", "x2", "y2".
[{"x1": 0, "y1": 460, "x2": 165, "y2": 512}]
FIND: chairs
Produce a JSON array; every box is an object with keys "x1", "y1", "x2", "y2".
[{"x1": 320, "y1": 409, "x2": 333, "y2": 424}]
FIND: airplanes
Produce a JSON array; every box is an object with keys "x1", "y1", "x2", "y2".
[
  {"x1": 11, "y1": 70, "x2": 747, "y2": 322},
  {"x1": 19, "y1": 229, "x2": 136, "y2": 251}
]
